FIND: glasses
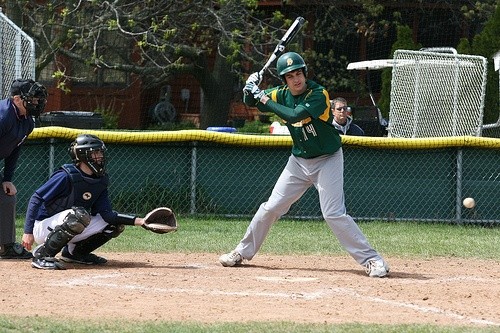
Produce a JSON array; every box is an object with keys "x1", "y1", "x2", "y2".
[{"x1": 334, "y1": 105, "x2": 347, "y2": 111}]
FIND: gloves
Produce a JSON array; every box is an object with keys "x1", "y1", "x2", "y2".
[
  {"x1": 243, "y1": 81, "x2": 267, "y2": 102},
  {"x1": 245, "y1": 71, "x2": 263, "y2": 87}
]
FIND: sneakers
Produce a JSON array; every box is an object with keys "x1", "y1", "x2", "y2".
[
  {"x1": 364, "y1": 259, "x2": 390, "y2": 277},
  {"x1": 219, "y1": 250, "x2": 245, "y2": 267},
  {"x1": 0, "y1": 241, "x2": 33, "y2": 259},
  {"x1": 61, "y1": 245, "x2": 98, "y2": 264},
  {"x1": 31, "y1": 257, "x2": 56, "y2": 269}
]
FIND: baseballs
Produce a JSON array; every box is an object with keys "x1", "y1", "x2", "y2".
[{"x1": 463, "y1": 198, "x2": 475, "y2": 208}]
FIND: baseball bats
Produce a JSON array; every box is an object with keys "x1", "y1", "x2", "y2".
[{"x1": 244, "y1": 16, "x2": 306, "y2": 96}]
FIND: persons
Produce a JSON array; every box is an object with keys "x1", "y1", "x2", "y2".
[
  {"x1": 330, "y1": 96, "x2": 364, "y2": 136},
  {"x1": 22, "y1": 134, "x2": 145, "y2": 270},
  {"x1": 0, "y1": 78, "x2": 48, "y2": 259},
  {"x1": 220, "y1": 52, "x2": 390, "y2": 278}
]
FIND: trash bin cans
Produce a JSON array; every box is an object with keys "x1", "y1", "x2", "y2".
[{"x1": 39, "y1": 110, "x2": 105, "y2": 129}]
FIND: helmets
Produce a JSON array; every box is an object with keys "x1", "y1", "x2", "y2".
[
  {"x1": 11, "y1": 78, "x2": 47, "y2": 98},
  {"x1": 277, "y1": 52, "x2": 309, "y2": 85},
  {"x1": 67, "y1": 134, "x2": 109, "y2": 177}
]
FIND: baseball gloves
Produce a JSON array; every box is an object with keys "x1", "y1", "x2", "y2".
[{"x1": 141, "y1": 207, "x2": 178, "y2": 234}]
